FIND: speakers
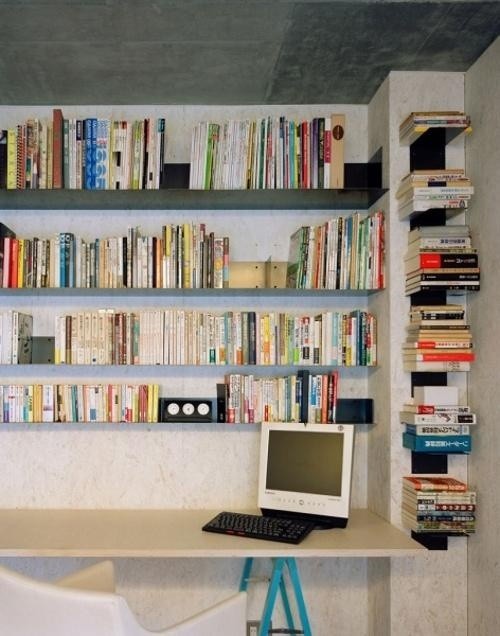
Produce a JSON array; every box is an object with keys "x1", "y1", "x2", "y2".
[{"x1": 158, "y1": 398, "x2": 213, "y2": 423}]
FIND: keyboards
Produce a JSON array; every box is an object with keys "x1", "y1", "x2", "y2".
[{"x1": 201, "y1": 511, "x2": 313, "y2": 545}]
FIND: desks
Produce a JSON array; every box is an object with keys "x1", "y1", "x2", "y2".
[{"x1": 1, "y1": 510, "x2": 430, "y2": 636}]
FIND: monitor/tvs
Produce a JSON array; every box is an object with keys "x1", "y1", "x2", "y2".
[{"x1": 257, "y1": 422, "x2": 355, "y2": 529}]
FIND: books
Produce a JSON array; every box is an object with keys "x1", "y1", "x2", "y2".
[
  {"x1": 397, "y1": 108, "x2": 483, "y2": 535},
  {"x1": 286, "y1": 209, "x2": 387, "y2": 288},
  {"x1": 188, "y1": 112, "x2": 345, "y2": 191},
  {"x1": 0, "y1": 222, "x2": 230, "y2": 289},
  {"x1": 0, "y1": 309, "x2": 376, "y2": 367},
  {"x1": 0, "y1": 109, "x2": 166, "y2": 190},
  {"x1": 1, "y1": 384, "x2": 159, "y2": 425},
  {"x1": 217, "y1": 370, "x2": 338, "y2": 423}
]
